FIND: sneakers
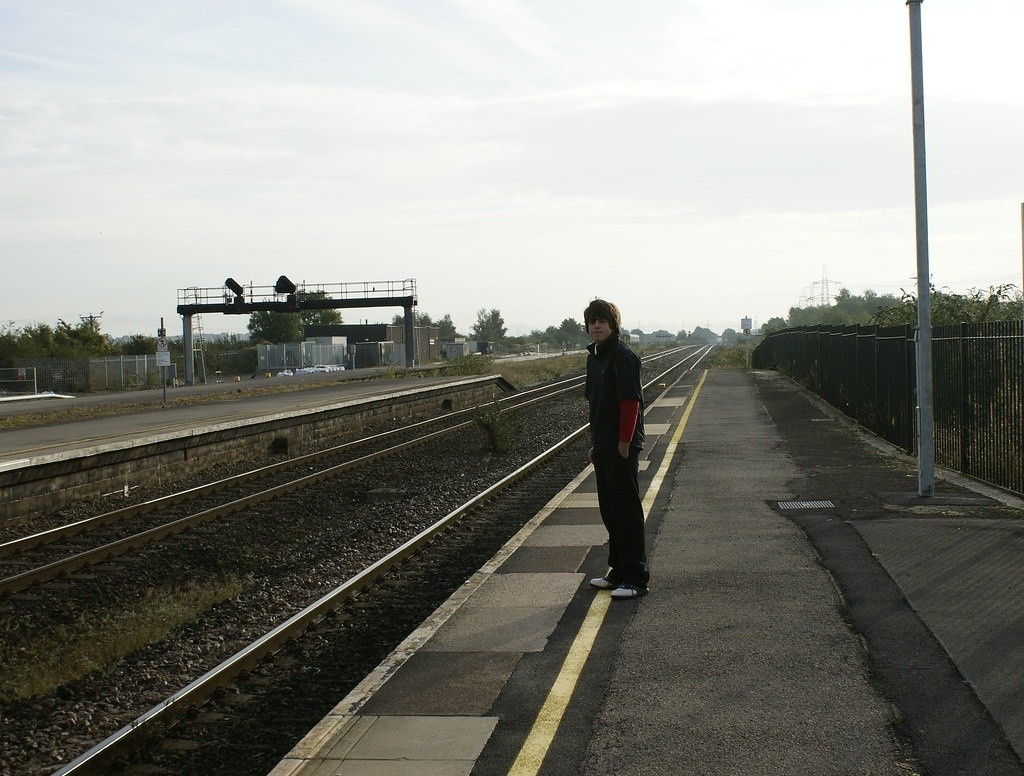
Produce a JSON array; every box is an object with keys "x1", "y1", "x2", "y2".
[
  {"x1": 589, "y1": 577, "x2": 618, "y2": 589},
  {"x1": 610, "y1": 581, "x2": 649, "y2": 600}
]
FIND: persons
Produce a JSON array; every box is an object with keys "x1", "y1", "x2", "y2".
[{"x1": 584, "y1": 300, "x2": 649, "y2": 599}]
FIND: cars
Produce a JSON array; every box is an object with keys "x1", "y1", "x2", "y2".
[{"x1": 277, "y1": 364, "x2": 346, "y2": 377}]
